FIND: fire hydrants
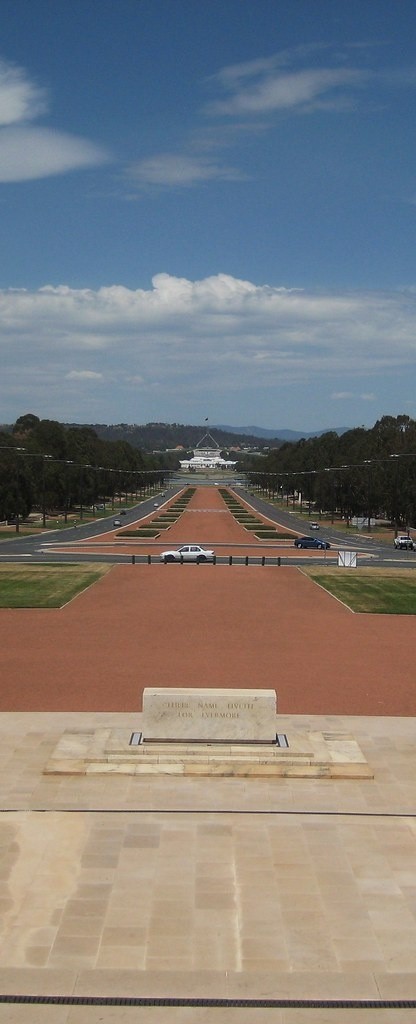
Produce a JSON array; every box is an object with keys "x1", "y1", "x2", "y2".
[{"x1": 74, "y1": 519, "x2": 77, "y2": 528}]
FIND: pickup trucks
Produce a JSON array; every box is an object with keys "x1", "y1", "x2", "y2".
[{"x1": 394, "y1": 536, "x2": 413, "y2": 550}]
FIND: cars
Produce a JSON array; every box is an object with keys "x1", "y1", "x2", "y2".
[
  {"x1": 309, "y1": 522, "x2": 320, "y2": 531},
  {"x1": 113, "y1": 519, "x2": 121, "y2": 527},
  {"x1": 294, "y1": 536, "x2": 331, "y2": 550},
  {"x1": 159, "y1": 545, "x2": 215, "y2": 563}
]
